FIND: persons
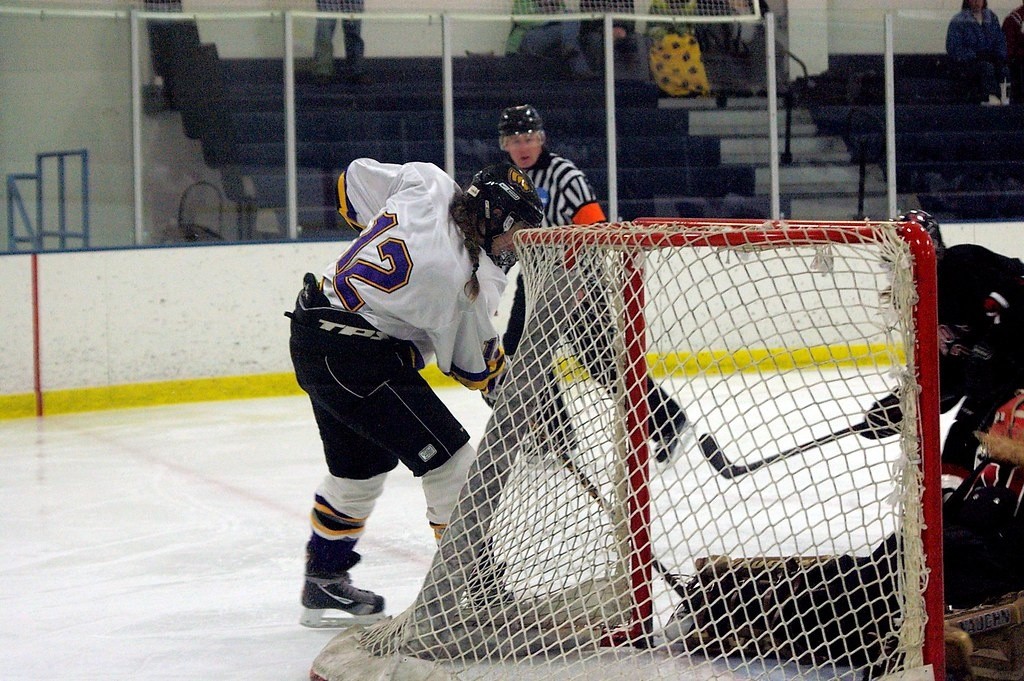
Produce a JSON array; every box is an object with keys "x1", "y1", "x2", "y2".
[
  {"x1": 309, "y1": 0, "x2": 373, "y2": 83},
  {"x1": 643, "y1": 0, "x2": 773, "y2": 95},
  {"x1": 859, "y1": 208, "x2": 1023, "y2": 611},
  {"x1": 941, "y1": 0, "x2": 1023, "y2": 104},
  {"x1": 501, "y1": 0, "x2": 641, "y2": 82},
  {"x1": 288, "y1": 104, "x2": 688, "y2": 631}
]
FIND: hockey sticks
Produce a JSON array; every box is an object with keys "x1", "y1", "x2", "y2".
[
  {"x1": 562, "y1": 453, "x2": 690, "y2": 600},
  {"x1": 699, "y1": 421, "x2": 866, "y2": 479}
]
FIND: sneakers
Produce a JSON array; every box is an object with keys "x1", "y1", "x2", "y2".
[
  {"x1": 522, "y1": 429, "x2": 581, "y2": 470},
  {"x1": 466, "y1": 561, "x2": 516, "y2": 610},
  {"x1": 655, "y1": 417, "x2": 697, "y2": 470},
  {"x1": 300, "y1": 571, "x2": 386, "y2": 628}
]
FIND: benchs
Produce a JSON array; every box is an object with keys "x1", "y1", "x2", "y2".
[{"x1": 224, "y1": 25, "x2": 1024, "y2": 238}]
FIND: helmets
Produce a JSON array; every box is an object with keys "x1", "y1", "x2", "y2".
[
  {"x1": 497, "y1": 104, "x2": 543, "y2": 137},
  {"x1": 462, "y1": 161, "x2": 544, "y2": 275},
  {"x1": 880, "y1": 209, "x2": 947, "y2": 265}
]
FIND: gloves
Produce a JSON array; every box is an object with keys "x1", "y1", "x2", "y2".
[{"x1": 859, "y1": 385, "x2": 905, "y2": 440}]
